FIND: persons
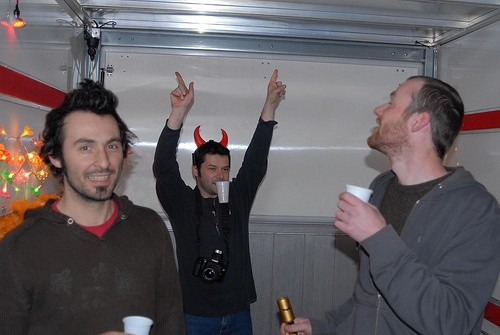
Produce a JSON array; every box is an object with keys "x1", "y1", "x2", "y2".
[
  {"x1": 153, "y1": 69, "x2": 288, "y2": 335},
  {"x1": 281, "y1": 76, "x2": 500, "y2": 335},
  {"x1": 0, "y1": 78, "x2": 186, "y2": 335}
]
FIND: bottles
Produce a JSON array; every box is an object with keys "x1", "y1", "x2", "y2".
[{"x1": 277, "y1": 297, "x2": 299, "y2": 335}]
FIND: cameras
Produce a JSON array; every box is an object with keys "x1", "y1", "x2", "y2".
[{"x1": 193, "y1": 249, "x2": 227, "y2": 283}]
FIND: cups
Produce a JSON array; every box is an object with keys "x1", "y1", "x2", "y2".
[
  {"x1": 215, "y1": 181, "x2": 230, "y2": 203},
  {"x1": 346, "y1": 185, "x2": 373, "y2": 202},
  {"x1": 122, "y1": 316, "x2": 153, "y2": 335}
]
